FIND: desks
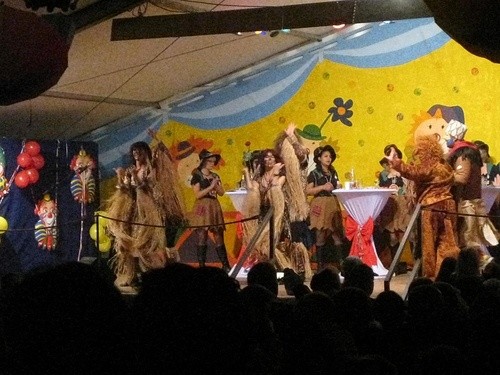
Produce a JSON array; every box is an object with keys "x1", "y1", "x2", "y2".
[
  {"x1": 224, "y1": 192, "x2": 283, "y2": 278},
  {"x1": 332, "y1": 189, "x2": 398, "y2": 278},
  {"x1": 480, "y1": 187, "x2": 500, "y2": 217}
]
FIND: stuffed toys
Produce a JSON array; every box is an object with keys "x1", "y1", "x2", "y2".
[{"x1": 384, "y1": 135, "x2": 460, "y2": 280}]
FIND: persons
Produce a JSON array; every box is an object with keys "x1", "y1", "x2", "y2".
[{"x1": 0, "y1": 119, "x2": 500, "y2": 375}]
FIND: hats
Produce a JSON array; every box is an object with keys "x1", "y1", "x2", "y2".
[
  {"x1": 445, "y1": 119, "x2": 467, "y2": 140},
  {"x1": 295, "y1": 124, "x2": 327, "y2": 141},
  {"x1": 198, "y1": 149, "x2": 221, "y2": 166},
  {"x1": 176, "y1": 141, "x2": 196, "y2": 160},
  {"x1": 475, "y1": 141, "x2": 489, "y2": 150},
  {"x1": 313, "y1": 145, "x2": 336, "y2": 164}
]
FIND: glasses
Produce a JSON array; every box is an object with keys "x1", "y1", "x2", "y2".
[{"x1": 207, "y1": 160, "x2": 216, "y2": 165}]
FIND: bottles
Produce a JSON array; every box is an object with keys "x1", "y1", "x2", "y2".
[{"x1": 241, "y1": 175, "x2": 245, "y2": 188}]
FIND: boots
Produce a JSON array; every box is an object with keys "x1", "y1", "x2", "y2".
[
  {"x1": 335, "y1": 244, "x2": 345, "y2": 260},
  {"x1": 215, "y1": 245, "x2": 231, "y2": 273},
  {"x1": 314, "y1": 245, "x2": 326, "y2": 274},
  {"x1": 196, "y1": 245, "x2": 207, "y2": 269}
]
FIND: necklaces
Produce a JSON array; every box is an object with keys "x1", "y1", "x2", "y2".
[{"x1": 202, "y1": 172, "x2": 211, "y2": 176}]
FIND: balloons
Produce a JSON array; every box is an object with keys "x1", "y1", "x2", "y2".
[{"x1": 0, "y1": 216, "x2": 8, "y2": 234}]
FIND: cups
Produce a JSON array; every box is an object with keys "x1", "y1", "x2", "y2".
[{"x1": 345, "y1": 182, "x2": 352, "y2": 190}]
